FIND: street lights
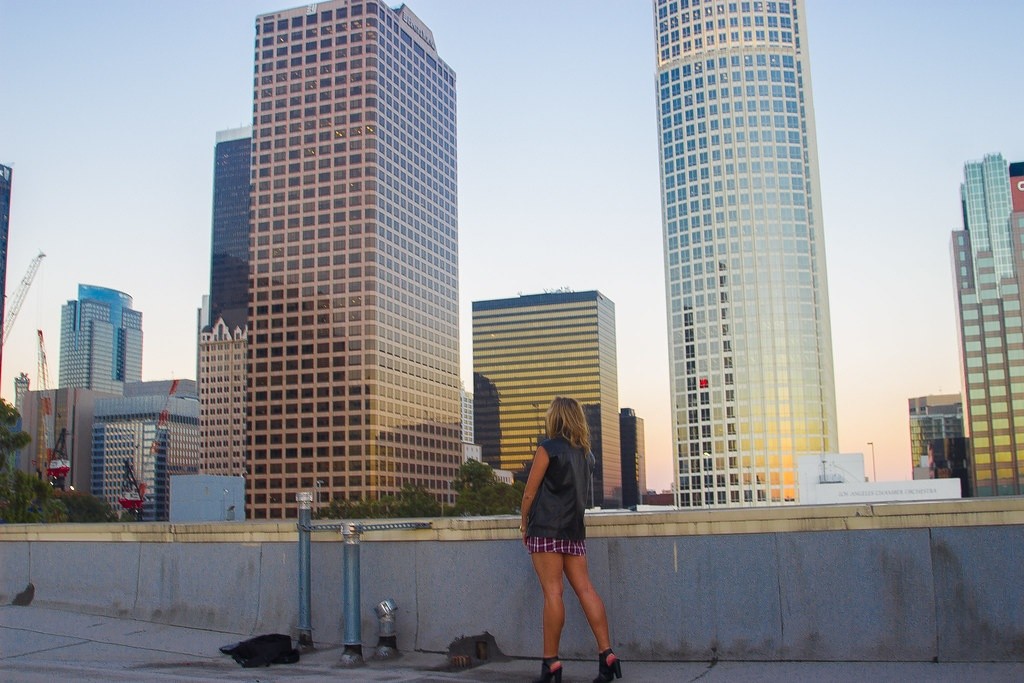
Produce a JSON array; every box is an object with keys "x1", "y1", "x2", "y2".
[{"x1": 867, "y1": 441, "x2": 875, "y2": 482}]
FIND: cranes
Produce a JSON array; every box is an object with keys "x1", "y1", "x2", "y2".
[
  {"x1": 118, "y1": 381, "x2": 179, "y2": 521},
  {"x1": 31, "y1": 330, "x2": 69, "y2": 495},
  {"x1": 3, "y1": 251, "x2": 47, "y2": 351}
]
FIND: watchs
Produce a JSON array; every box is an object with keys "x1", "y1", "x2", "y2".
[{"x1": 520, "y1": 526, "x2": 527, "y2": 532}]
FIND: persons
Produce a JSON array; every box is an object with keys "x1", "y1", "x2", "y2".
[{"x1": 520, "y1": 397, "x2": 622, "y2": 683}]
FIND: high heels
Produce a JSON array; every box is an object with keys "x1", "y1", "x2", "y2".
[
  {"x1": 535, "y1": 656, "x2": 564, "y2": 683},
  {"x1": 592, "y1": 648, "x2": 622, "y2": 683}
]
